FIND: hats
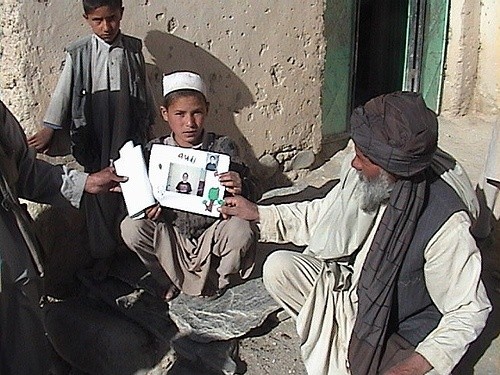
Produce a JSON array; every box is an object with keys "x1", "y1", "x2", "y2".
[{"x1": 162, "y1": 72, "x2": 207, "y2": 99}]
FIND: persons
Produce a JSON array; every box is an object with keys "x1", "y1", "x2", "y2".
[
  {"x1": 26, "y1": 0, "x2": 159, "y2": 282},
  {"x1": 217, "y1": 91, "x2": 493, "y2": 375},
  {"x1": 205, "y1": 156, "x2": 217, "y2": 171},
  {"x1": 175, "y1": 172, "x2": 192, "y2": 194},
  {"x1": 119, "y1": 70, "x2": 262, "y2": 301},
  {"x1": 0, "y1": 100, "x2": 129, "y2": 375}
]
